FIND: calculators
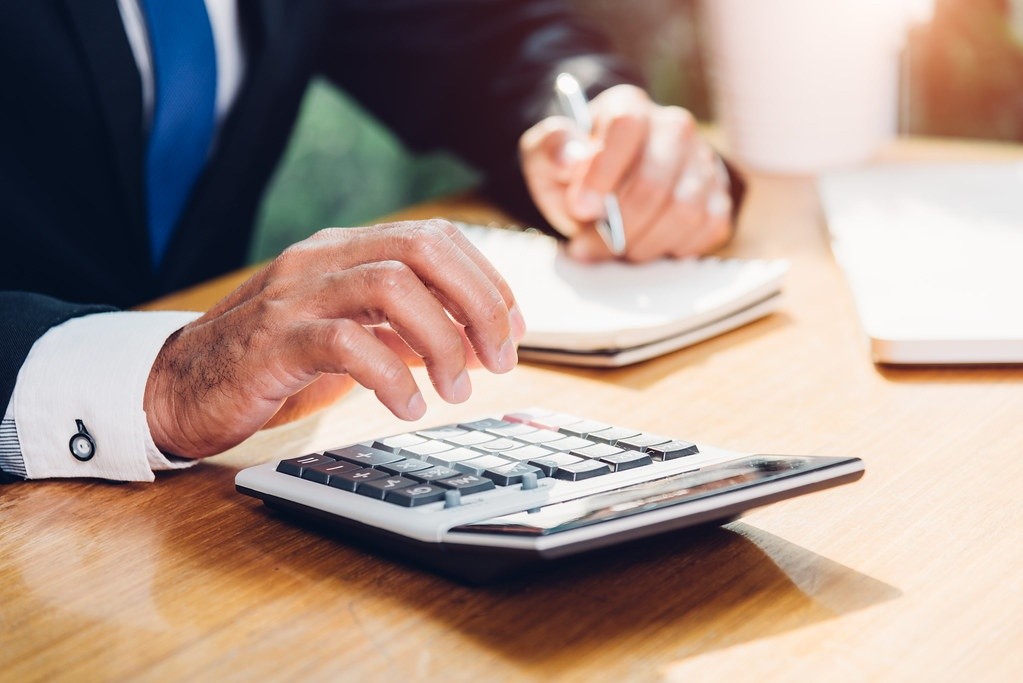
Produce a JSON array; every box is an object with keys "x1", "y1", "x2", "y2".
[{"x1": 233, "y1": 403, "x2": 867, "y2": 589}]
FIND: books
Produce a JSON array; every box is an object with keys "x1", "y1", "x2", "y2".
[
  {"x1": 442, "y1": 221, "x2": 789, "y2": 370},
  {"x1": 818, "y1": 166, "x2": 1023, "y2": 364}
]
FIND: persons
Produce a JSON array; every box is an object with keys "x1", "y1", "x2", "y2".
[{"x1": 0, "y1": 0, "x2": 745, "y2": 485}]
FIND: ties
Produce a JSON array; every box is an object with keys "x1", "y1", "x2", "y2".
[{"x1": 138, "y1": 1, "x2": 218, "y2": 267}]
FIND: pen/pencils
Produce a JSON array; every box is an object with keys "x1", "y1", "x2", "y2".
[{"x1": 555, "y1": 71, "x2": 626, "y2": 258}]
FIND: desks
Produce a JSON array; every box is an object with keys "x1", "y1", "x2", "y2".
[{"x1": 0, "y1": 121, "x2": 1023, "y2": 683}]
